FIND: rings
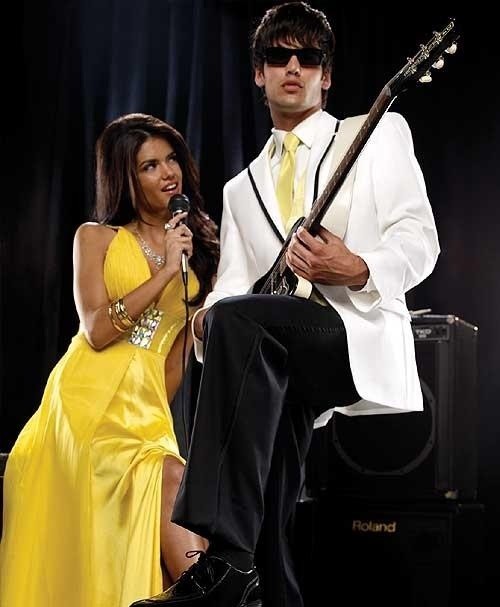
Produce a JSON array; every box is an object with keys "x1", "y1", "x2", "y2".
[{"x1": 164, "y1": 223, "x2": 173, "y2": 230}]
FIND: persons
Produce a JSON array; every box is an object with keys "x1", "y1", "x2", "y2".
[
  {"x1": 128, "y1": 1, "x2": 441, "y2": 604},
  {"x1": 0, "y1": 109, "x2": 219, "y2": 607}
]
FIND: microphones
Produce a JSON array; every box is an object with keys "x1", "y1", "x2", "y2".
[{"x1": 168, "y1": 191, "x2": 194, "y2": 288}]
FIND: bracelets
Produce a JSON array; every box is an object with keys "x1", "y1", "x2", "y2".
[{"x1": 108, "y1": 298, "x2": 134, "y2": 333}]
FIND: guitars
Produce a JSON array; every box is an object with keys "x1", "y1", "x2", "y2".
[{"x1": 245, "y1": 18, "x2": 463, "y2": 298}]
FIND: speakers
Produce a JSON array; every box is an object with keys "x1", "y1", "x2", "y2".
[{"x1": 302, "y1": 312, "x2": 477, "y2": 504}]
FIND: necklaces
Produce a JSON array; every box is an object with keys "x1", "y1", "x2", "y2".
[{"x1": 129, "y1": 221, "x2": 166, "y2": 265}]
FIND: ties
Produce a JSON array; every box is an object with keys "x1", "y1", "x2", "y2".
[{"x1": 275, "y1": 132, "x2": 300, "y2": 235}]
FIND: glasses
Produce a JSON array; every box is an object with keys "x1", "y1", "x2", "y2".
[{"x1": 264, "y1": 46, "x2": 324, "y2": 66}]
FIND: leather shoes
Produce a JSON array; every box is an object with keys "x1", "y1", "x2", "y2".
[{"x1": 128, "y1": 551, "x2": 263, "y2": 607}]
